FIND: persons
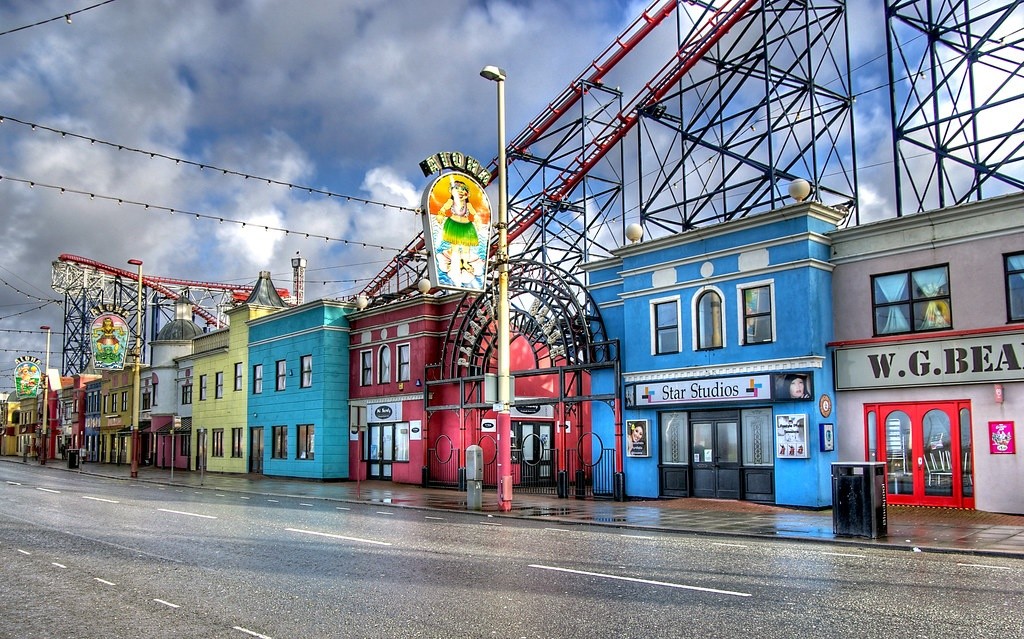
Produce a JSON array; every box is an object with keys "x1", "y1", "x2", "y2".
[
  {"x1": 783, "y1": 374, "x2": 810, "y2": 398},
  {"x1": 627, "y1": 422, "x2": 646, "y2": 455}
]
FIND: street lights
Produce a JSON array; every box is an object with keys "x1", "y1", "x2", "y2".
[
  {"x1": 131, "y1": 258, "x2": 144, "y2": 479},
  {"x1": 40, "y1": 325, "x2": 51, "y2": 466},
  {"x1": 480, "y1": 64, "x2": 513, "y2": 512}
]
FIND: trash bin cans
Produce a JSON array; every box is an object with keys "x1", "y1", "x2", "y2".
[
  {"x1": 830, "y1": 461, "x2": 888, "y2": 538},
  {"x1": 65, "y1": 449, "x2": 79, "y2": 469}
]
